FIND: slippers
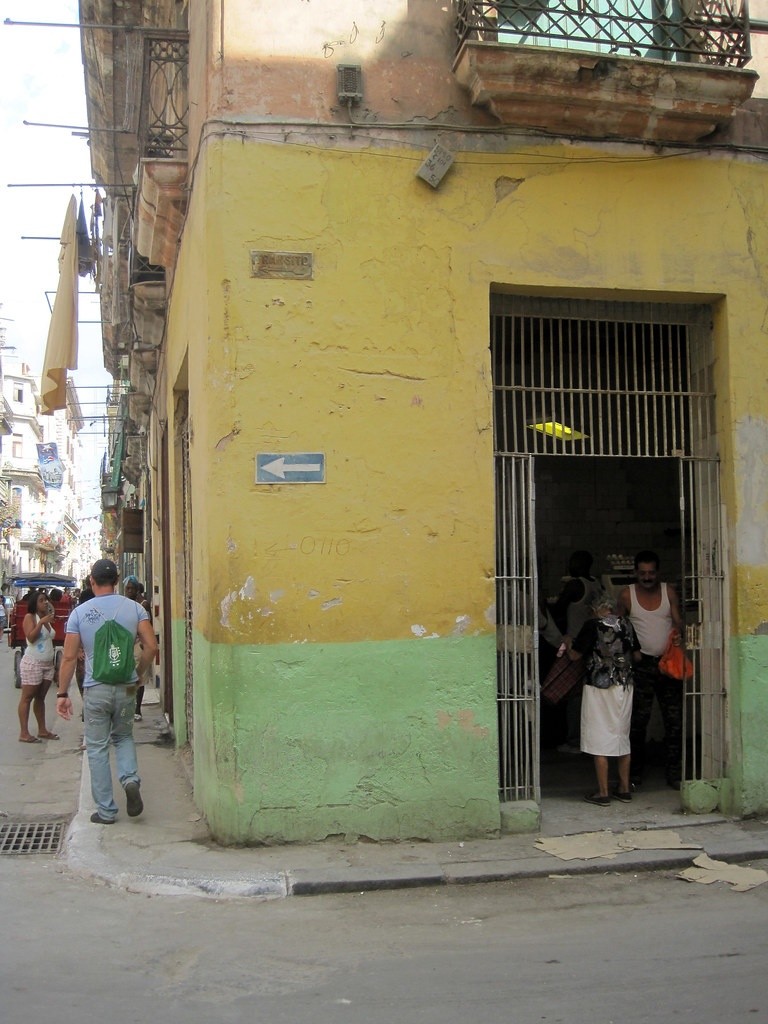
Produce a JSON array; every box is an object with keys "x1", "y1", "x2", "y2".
[
  {"x1": 20, "y1": 736, "x2": 42, "y2": 743},
  {"x1": 38, "y1": 732, "x2": 59, "y2": 740}
]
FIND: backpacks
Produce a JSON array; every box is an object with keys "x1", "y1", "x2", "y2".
[{"x1": 91, "y1": 619, "x2": 136, "y2": 685}]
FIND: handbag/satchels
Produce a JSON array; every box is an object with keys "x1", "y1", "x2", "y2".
[
  {"x1": 542, "y1": 653, "x2": 583, "y2": 702},
  {"x1": 661, "y1": 631, "x2": 693, "y2": 680}
]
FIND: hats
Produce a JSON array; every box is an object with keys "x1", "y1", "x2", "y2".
[{"x1": 123, "y1": 574, "x2": 138, "y2": 587}]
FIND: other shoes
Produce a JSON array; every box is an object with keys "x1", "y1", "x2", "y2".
[
  {"x1": 90, "y1": 812, "x2": 114, "y2": 825},
  {"x1": 134, "y1": 713, "x2": 142, "y2": 721},
  {"x1": 125, "y1": 783, "x2": 143, "y2": 816},
  {"x1": 667, "y1": 779, "x2": 681, "y2": 790},
  {"x1": 584, "y1": 793, "x2": 610, "y2": 806},
  {"x1": 611, "y1": 781, "x2": 639, "y2": 792},
  {"x1": 610, "y1": 790, "x2": 632, "y2": 803},
  {"x1": 80, "y1": 745, "x2": 86, "y2": 750}
]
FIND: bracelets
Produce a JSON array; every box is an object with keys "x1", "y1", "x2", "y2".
[{"x1": 57, "y1": 692, "x2": 68, "y2": 698}]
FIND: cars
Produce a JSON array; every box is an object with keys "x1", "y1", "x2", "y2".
[{"x1": 5, "y1": 595, "x2": 16, "y2": 626}]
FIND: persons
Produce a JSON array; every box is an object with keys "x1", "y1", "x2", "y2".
[
  {"x1": 22, "y1": 587, "x2": 62, "y2": 602},
  {"x1": 18, "y1": 593, "x2": 59, "y2": 743},
  {"x1": 0, "y1": 595, "x2": 7, "y2": 642},
  {"x1": 536, "y1": 551, "x2": 689, "y2": 806},
  {"x1": 57, "y1": 559, "x2": 158, "y2": 824},
  {"x1": 65, "y1": 575, "x2": 153, "y2": 721}
]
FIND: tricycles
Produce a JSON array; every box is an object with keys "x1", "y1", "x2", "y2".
[{"x1": 8, "y1": 573, "x2": 77, "y2": 690}]
